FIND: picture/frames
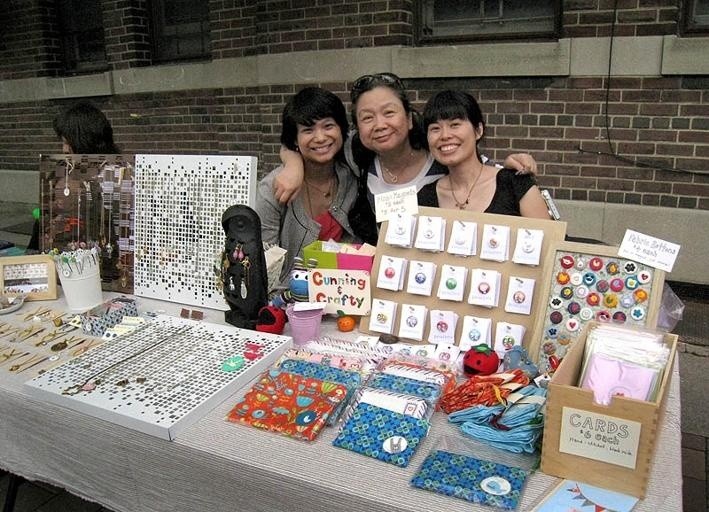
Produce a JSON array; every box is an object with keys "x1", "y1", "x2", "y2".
[
  {"x1": 527, "y1": 237, "x2": 666, "y2": 375},
  {"x1": 0, "y1": 253, "x2": 59, "y2": 303}
]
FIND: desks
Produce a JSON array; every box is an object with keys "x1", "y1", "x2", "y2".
[{"x1": 0, "y1": 273, "x2": 683, "y2": 512}]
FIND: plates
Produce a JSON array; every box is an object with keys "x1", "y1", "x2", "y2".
[{"x1": 0, "y1": 297, "x2": 24, "y2": 316}]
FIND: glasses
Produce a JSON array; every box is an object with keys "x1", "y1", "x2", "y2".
[{"x1": 351, "y1": 73, "x2": 404, "y2": 97}]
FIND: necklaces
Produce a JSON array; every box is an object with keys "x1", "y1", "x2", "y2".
[
  {"x1": 0, "y1": 305, "x2": 194, "y2": 396},
  {"x1": 41, "y1": 159, "x2": 129, "y2": 287},
  {"x1": 380, "y1": 149, "x2": 415, "y2": 183},
  {"x1": 303, "y1": 176, "x2": 332, "y2": 198},
  {"x1": 449, "y1": 164, "x2": 483, "y2": 210}
]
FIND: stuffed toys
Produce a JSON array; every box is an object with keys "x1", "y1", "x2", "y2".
[
  {"x1": 463, "y1": 343, "x2": 499, "y2": 377},
  {"x1": 268, "y1": 257, "x2": 317, "y2": 322}
]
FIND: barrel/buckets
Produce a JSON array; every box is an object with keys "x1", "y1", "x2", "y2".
[{"x1": 53, "y1": 243, "x2": 104, "y2": 314}]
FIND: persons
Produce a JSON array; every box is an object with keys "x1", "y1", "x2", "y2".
[
  {"x1": 256, "y1": 87, "x2": 358, "y2": 283},
  {"x1": 27, "y1": 104, "x2": 123, "y2": 283},
  {"x1": 416, "y1": 90, "x2": 550, "y2": 220},
  {"x1": 274, "y1": 74, "x2": 538, "y2": 246}
]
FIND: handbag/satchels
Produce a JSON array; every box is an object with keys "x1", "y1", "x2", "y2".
[{"x1": 348, "y1": 193, "x2": 378, "y2": 246}]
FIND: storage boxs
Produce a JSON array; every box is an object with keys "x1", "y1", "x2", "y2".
[{"x1": 540, "y1": 320, "x2": 678, "y2": 500}]
